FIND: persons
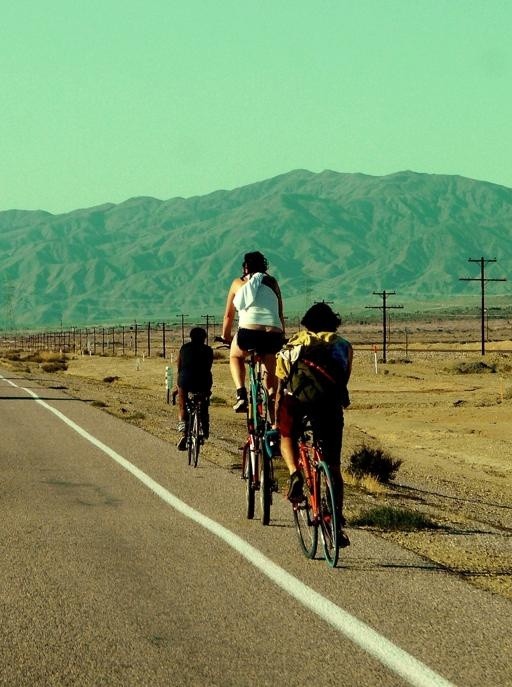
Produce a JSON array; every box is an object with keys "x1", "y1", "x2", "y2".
[
  {"x1": 177, "y1": 328, "x2": 213, "y2": 450},
  {"x1": 272, "y1": 302, "x2": 353, "y2": 548},
  {"x1": 221, "y1": 252, "x2": 285, "y2": 456}
]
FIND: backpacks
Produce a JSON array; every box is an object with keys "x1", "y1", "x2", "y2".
[{"x1": 290, "y1": 360, "x2": 337, "y2": 401}]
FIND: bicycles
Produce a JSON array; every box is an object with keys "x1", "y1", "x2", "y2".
[
  {"x1": 213, "y1": 336, "x2": 342, "y2": 568},
  {"x1": 172, "y1": 389, "x2": 204, "y2": 468}
]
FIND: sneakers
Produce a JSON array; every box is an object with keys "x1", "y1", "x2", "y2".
[
  {"x1": 287, "y1": 471, "x2": 303, "y2": 503},
  {"x1": 177, "y1": 434, "x2": 186, "y2": 451},
  {"x1": 232, "y1": 395, "x2": 248, "y2": 409}
]
